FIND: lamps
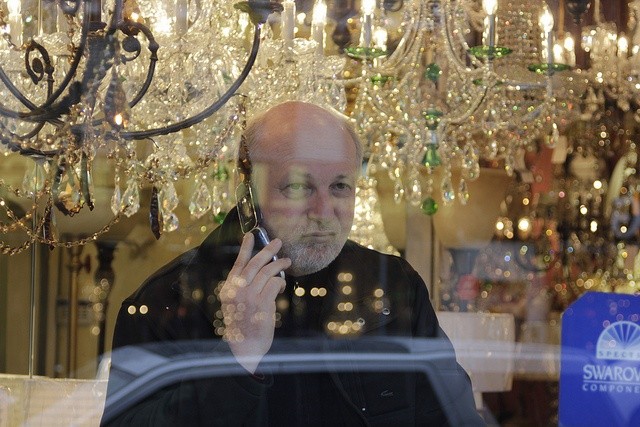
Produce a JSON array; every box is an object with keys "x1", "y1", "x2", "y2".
[
  {"x1": 92, "y1": 210, "x2": 139, "y2": 378},
  {"x1": 1, "y1": 0, "x2": 349, "y2": 262},
  {"x1": 43, "y1": 185, "x2": 116, "y2": 377},
  {"x1": 281, "y1": 0, "x2": 588, "y2": 217},
  {"x1": 570, "y1": 2, "x2": 640, "y2": 112},
  {"x1": 436, "y1": 307, "x2": 518, "y2": 412}
]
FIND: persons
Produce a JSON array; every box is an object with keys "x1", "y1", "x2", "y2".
[{"x1": 97, "y1": 101, "x2": 488, "y2": 424}]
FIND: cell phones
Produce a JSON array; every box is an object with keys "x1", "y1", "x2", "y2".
[{"x1": 235, "y1": 181, "x2": 287, "y2": 279}]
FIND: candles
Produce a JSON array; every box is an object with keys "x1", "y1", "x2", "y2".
[
  {"x1": 282, "y1": 1, "x2": 296, "y2": 40},
  {"x1": 480, "y1": 0, "x2": 499, "y2": 47},
  {"x1": 305, "y1": 0, "x2": 328, "y2": 52},
  {"x1": 539, "y1": 7, "x2": 556, "y2": 61},
  {"x1": 359, "y1": 0, "x2": 377, "y2": 46}
]
